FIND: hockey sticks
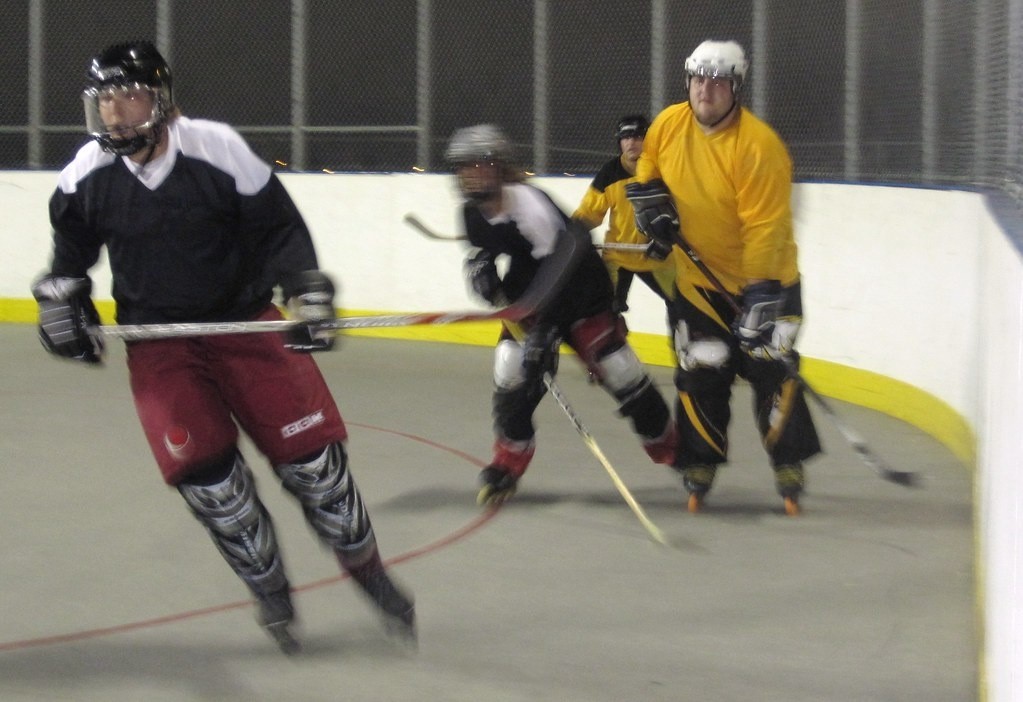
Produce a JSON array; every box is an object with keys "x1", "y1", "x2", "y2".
[
  {"x1": 678, "y1": 234, "x2": 929, "y2": 492},
  {"x1": 84, "y1": 231, "x2": 578, "y2": 339},
  {"x1": 496, "y1": 298, "x2": 704, "y2": 559},
  {"x1": 403, "y1": 213, "x2": 648, "y2": 254}
]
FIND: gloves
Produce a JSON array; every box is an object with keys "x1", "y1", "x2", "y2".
[
  {"x1": 284, "y1": 271, "x2": 336, "y2": 353},
  {"x1": 522, "y1": 322, "x2": 562, "y2": 378},
  {"x1": 730, "y1": 282, "x2": 785, "y2": 351},
  {"x1": 472, "y1": 260, "x2": 500, "y2": 300},
  {"x1": 626, "y1": 179, "x2": 681, "y2": 244},
  {"x1": 29, "y1": 274, "x2": 103, "y2": 363}
]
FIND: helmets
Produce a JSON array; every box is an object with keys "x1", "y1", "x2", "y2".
[
  {"x1": 686, "y1": 40, "x2": 748, "y2": 94},
  {"x1": 615, "y1": 115, "x2": 649, "y2": 140},
  {"x1": 88, "y1": 40, "x2": 170, "y2": 156},
  {"x1": 445, "y1": 123, "x2": 513, "y2": 160}
]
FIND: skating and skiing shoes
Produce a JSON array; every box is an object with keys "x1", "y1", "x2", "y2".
[
  {"x1": 475, "y1": 445, "x2": 536, "y2": 503},
  {"x1": 250, "y1": 582, "x2": 299, "y2": 653},
  {"x1": 685, "y1": 462, "x2": 717, "y2": 511},
  {"x1": 361, "y1": 567, "x2": 417, "y2": 641},
  {"x1": 774, "y1": 460, "x2": 804, "y2": 514}
]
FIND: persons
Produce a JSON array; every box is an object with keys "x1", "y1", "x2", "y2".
[
  {"x1": 568, "y1": 116, "x2": 676, "y2": 385},
  {"x1": 31, "y1": 42, "x2": 421, "y2": 655},
  {"x1": 444, "y1": 124, "x2": 688, "y2": 508},
  {"x1": 623, "y1": 37, "x2": 820, "y2": 513}
]
